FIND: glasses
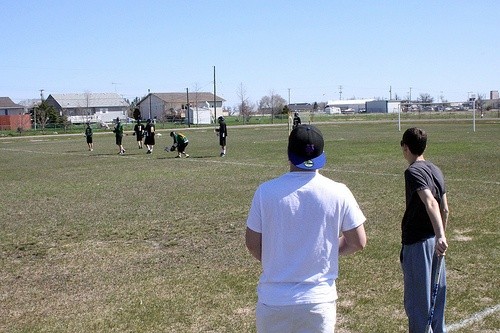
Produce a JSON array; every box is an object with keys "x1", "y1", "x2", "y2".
[{"x1": 400, "y1": 139, "x2": 405, "y2": 146}]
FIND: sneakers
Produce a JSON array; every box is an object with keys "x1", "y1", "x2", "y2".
[
  {"x1": 118, "y1": 149, "x2": 126, "y2": 154},
  {"x1": 89, "y1": 148, "x2": 94, "y2": 151}
]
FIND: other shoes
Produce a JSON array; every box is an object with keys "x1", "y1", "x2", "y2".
[
  {"x1": 175, "y1": 156, "x2": 181, "y2": 158},
  {"x1": 138, "y1": 145, "x2": 143, "y2": 149},
  {"x1": 220, "y1": 153, "x2": 226, "y2": 157},
  {"x1": 147, "y1": 149, "x2": 152, "y2": 153},
  {"x1": 184, "y1": 154, "x2": 189, "y2": 158}
]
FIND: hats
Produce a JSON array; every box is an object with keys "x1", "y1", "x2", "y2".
[{"x1": 288, "y1": 124, "x2": 325, "y2": 170}]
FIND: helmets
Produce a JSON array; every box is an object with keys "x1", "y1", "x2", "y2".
[
  {"x1": 147, "y1": 119, "x2": 152, "y2": 123},
  {"x1": 116, "y1": 117, "x2": 120, "y2": 121},
  {"x1": 137, "y1": 120, "x2": 141, "y2": 123},
  {"x1": 294, "y1": 112, "x2": 299, "y2": 116},
  {"x1": 170, "y1": 131, "x2": 176, "y2": 137},
  {"x1": 218, "y1": 116, "x2": 225, "y2": 120}
]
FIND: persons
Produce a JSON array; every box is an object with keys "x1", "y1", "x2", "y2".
[
  {"x1": 170, "y1": 132, "x2": 189, "y2": 158},
  {"x1": 215, "y1": 116, "x2": 227, "y2": 157},
  {"x1": 246, "y1": 124, "x2": 367, "y2": 333},
  {"x1": 85, "y1": 122, "x2": 94, "y2": 152},
  {"x1": 134, "y1": 119, "x2": 155, "y2": 154},
  {"x1": 292, "y1": 112, "x2": 301, "y2": 129},
  {"x1": 111, "y1": 117, "x2": 126, "y2": 155},
  {"x1": 399, "y1": 128, "x2": 448, "y2": 333}
]
